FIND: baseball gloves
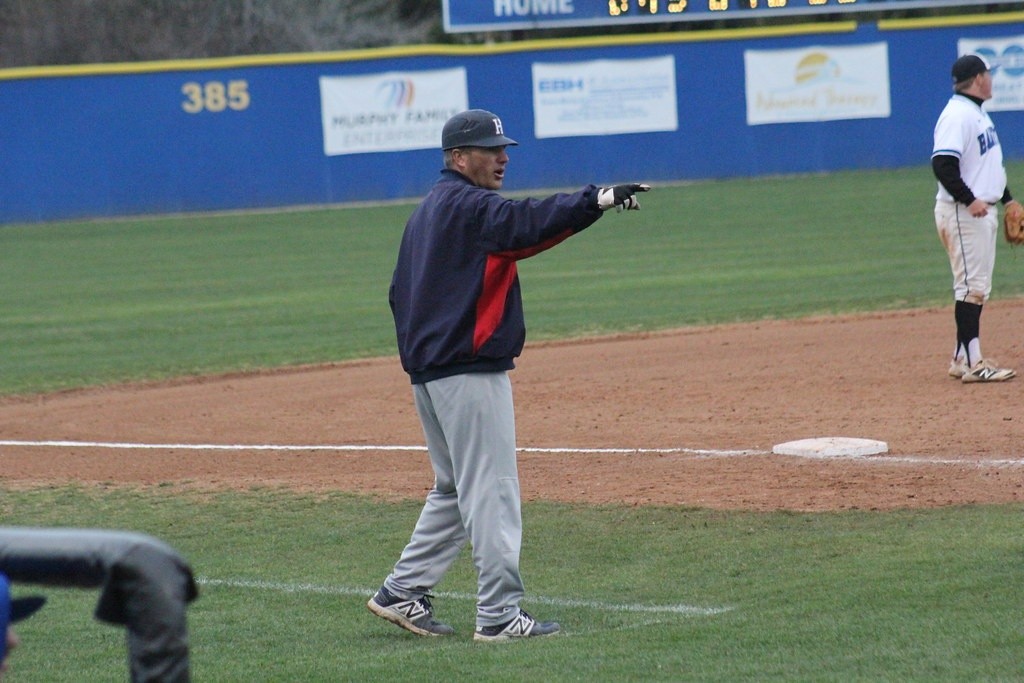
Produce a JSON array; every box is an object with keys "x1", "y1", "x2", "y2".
[{"x1": 1004, "y1": 201, "x2": 1024, "y2": 245}]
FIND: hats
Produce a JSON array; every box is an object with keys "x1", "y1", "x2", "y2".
[
  {"x1": 952, "y1": 55, "x2": 1000, "y2": 84},
  {"x1": 442, "y1": 110, "x2": 518, "y2": 148}
]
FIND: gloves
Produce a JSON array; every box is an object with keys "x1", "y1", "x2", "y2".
[{"x1": 596, "y1": 183, "x2": 650, "y2": 213}]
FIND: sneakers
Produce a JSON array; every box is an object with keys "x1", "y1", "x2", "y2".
[
  {"x1": 366, "y1": 585, "x2": 455, "y2": 638},
  {"x1": 474, "y1": 609, "x2": 561, "y2": 643},
  {"x1": 948, "y1": 359, "x2": 967, "y2": 376},
  {"x1": 962, "y1": 360, "x2": 1017, "y2": 384}
]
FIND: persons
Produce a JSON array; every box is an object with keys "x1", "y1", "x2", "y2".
[
  {"x1": 934, "y1": 55, "x2": 1024, "y2": 383},
  {"x1": 0, "y1": 575, "x2": 46, "y2": 672},
  {"x1": 368, "y1": 109, "x2": 651, "y2": 644}
]
